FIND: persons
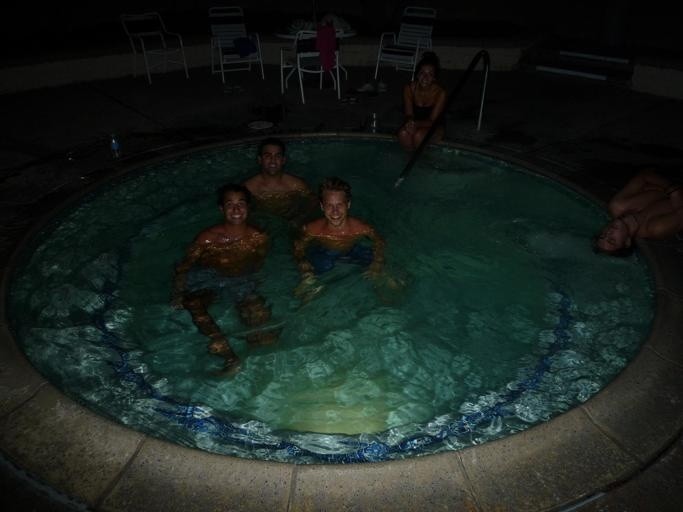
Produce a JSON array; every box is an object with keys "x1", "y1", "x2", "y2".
[
  {"x1": 295, "y1": 176, "x2": 404, "y2": 306},
  {"x1": 590, "y1": 169, "x2": 683, "y2": 257},
  {"x1": 241, "y1": 138, "x2": 320, "y2": 217},
  {"x1": 172, "y1": 183, "x2": 269, "y2": 382},
  {"x1": 399, "y1": 51, "x2": 448, "y2": 150}
]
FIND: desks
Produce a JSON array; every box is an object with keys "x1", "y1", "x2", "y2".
[{"x1": 274, "y1": 29, "x2": 359, "y2": 88}]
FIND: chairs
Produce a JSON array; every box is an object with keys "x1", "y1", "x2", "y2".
[
  {"x1": 207, "y1": 5, "x2": 266, "y2": 85},
  {"x1": 277, "y1": 28, "x2": 346, "y2": 105},
  {"x1": 120, "y1": 11, "x2": 192, "y2": 86},
  {"x1": 373, "y1": 5, "x2": 435, "y2": 83}
]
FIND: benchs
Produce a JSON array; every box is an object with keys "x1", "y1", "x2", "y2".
[{"x1": 520, "y1": 50, "x2": 635, "y2": 98}]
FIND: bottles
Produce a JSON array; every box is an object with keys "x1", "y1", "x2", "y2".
[
  {"x1": 368, "y1": 112, "x2": 377, "y2": 135},
  {"x1": 108, "y1": 133, "x2": 121, "y2": 159}
]
